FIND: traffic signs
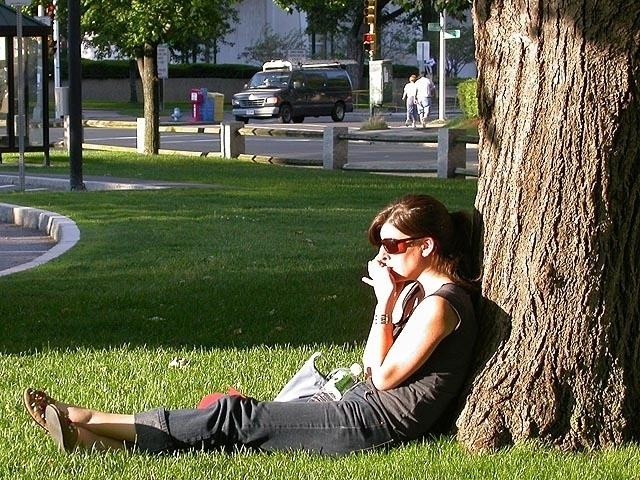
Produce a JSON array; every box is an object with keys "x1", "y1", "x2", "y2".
[{"x1": 427, "y1": 12, "x2": 461, "y2": 39}]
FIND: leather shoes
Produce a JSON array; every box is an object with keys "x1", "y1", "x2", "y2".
[{"x1": 24, "y1": 387, "x2": 75, "y2": 455}]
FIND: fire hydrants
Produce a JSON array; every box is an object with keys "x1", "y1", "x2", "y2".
[{"x1": 171, "y1": 107, "x2": 183, "y2": 121}]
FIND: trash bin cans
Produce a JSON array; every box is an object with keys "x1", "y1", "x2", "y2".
[
  {"x1": 200, "y1": 95, "x2": 214, "y2": 121},
  {"x1": 207, "y1": 92, "x2": 224, "y2": 122},
  {"x1": 190, "y1": 88, "x2": 209, "y2": 121}
]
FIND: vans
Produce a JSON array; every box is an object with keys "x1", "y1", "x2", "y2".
[{"x1": 232, "y1": 59, "x2": 353, "y2": 124}]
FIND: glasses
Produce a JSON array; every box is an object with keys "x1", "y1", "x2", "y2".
[{"x1": 381, "y1": 238, "x2": 430, "y2": 254}]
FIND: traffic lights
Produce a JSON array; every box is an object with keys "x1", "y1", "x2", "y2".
[{"x1": 363, "y1": 0, "x2": 377, "y2": 51}]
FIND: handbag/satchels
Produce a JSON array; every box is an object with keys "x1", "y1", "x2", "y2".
[{"x1": 274, "y1": 352, "x2": 329, "y2": 402}]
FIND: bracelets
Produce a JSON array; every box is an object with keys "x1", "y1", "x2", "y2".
[{"x1": 374, "y1": 313, "x2": 391, "y2": 324}]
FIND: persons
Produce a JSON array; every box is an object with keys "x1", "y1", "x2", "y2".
[
  {"x1": 413, "y1": 72, "x2": 435, "y2": 128},
  {"x1": 402, "y1": 74, "x2": 418, "y2": 128},
  {"x1": 23, "y1": 195, "x2": 484, "y2": 458}
]
